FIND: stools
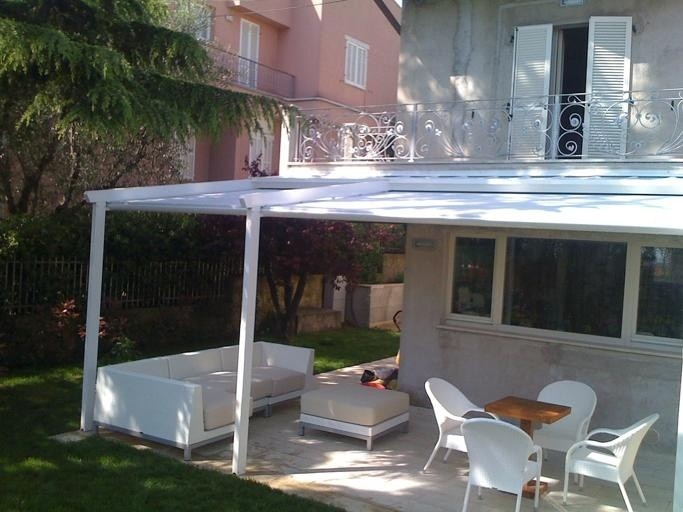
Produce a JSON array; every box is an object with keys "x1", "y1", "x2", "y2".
[{"x1": 298, "y1": 382, "x2": 409, "y2": 450}]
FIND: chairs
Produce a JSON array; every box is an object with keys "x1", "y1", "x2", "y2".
[
  {"x1": 460, "y1": 418, "x2": 542, "y2": 512},
  {"x1": 423, "y1": 377, "x2": 499, "y2": 471},
  {"x1": 562, "y1": 413, "x2": 659, "y2": 512},
  {"x1": 533, "y1": 380, "x2": 597, "y2": 485}
]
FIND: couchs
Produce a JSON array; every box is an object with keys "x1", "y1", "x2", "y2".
[{"x1": 92, "y1": 341, "x2": 315, "y2": 461}]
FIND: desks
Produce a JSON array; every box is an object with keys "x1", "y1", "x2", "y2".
[{"x1": 485, "y1": 395, "x2": 571, "y2": 499}]
